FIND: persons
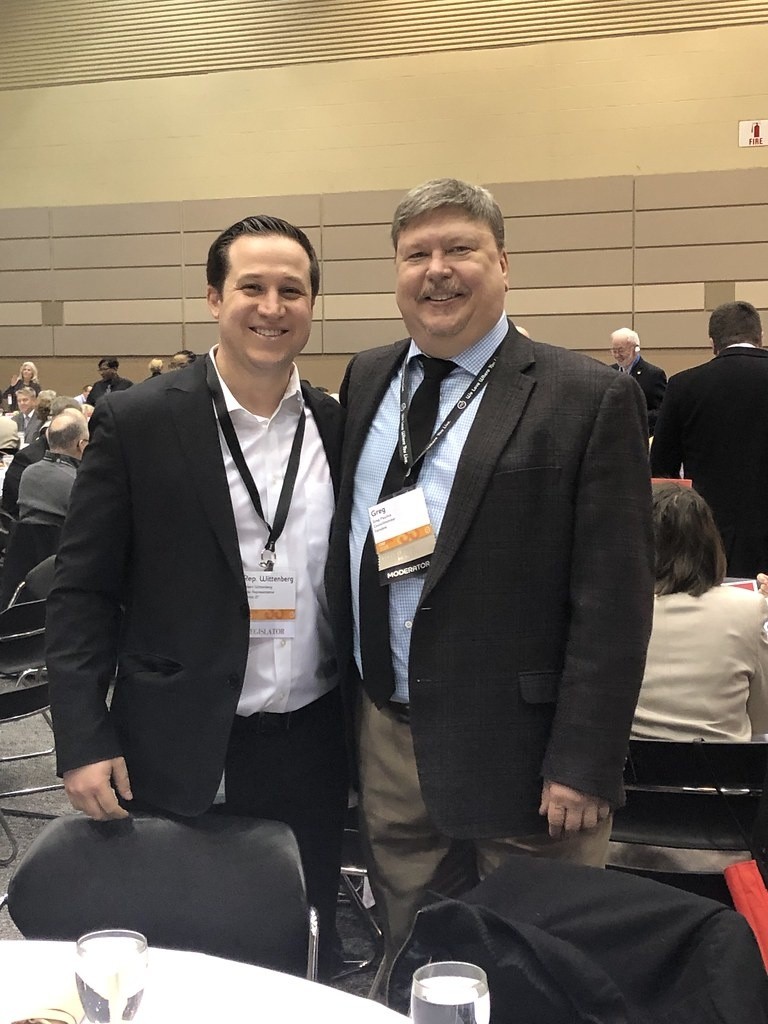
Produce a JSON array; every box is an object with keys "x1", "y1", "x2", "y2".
[
  {"x1": 604, "y1": 326, "x2": 668, "y2": 451},
  {"x1": 17, "y1": 407, "x2": 90, "y2": 531},
  {"x1": 1, "y1": 396, "x2": 83, "y2": 527},
  {"x1": 628, "y1": 480, "x2": 768, "y2": 851},
  {"x1": 649, "y1": 299, "x2": 768, "y2": 581},
  {"x1": 0, "y1": 349, "x2": 346, "y2": 458},
  {"x1": 324, "y1": 175, "x2": 652, "y2": 1000},
  {"x1": 43, "y1": 213, "x2": 351, "y2": 986}
]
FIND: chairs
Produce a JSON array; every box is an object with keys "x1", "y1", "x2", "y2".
[
  {"x1": 9, "y1": 554, "x2": 58, "y2": 607},
  {"x1": 603, "y1": 737, "x2": 768, "y2": 910},
  {"x1": 0, "y1": 810, "x2": 319, "y2": 987},
  {"x1": 0, "y1": 507, "x2": 17, "y2": 570},
  {"x1": 0, "y1": 598, "x2": 68, "y2": 867},
  {"x1": 316, "y1": 824, "x2": 383, "y2": 978},
  {"x1": 390, "y1": 854, "x2": 767, "y2": 1024},
  {"x1": 2, "y1": 522, "x2": 61, "y2": 607}
]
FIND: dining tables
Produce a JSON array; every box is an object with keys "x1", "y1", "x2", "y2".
[{"x1": 0, "y1": 936, "x2": 413, "y2": 1024}]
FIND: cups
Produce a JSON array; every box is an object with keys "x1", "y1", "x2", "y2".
[
  {"x1": 411, "y1": 961, "x2": 491, "y2": 1024},
  {"x1": 76, "y1": 930, "x2": 148, "y2": 1022}
]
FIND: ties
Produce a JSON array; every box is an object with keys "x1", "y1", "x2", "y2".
[{"x1": 358, "y1": 354, "x2": 457, "y2": 709}]
